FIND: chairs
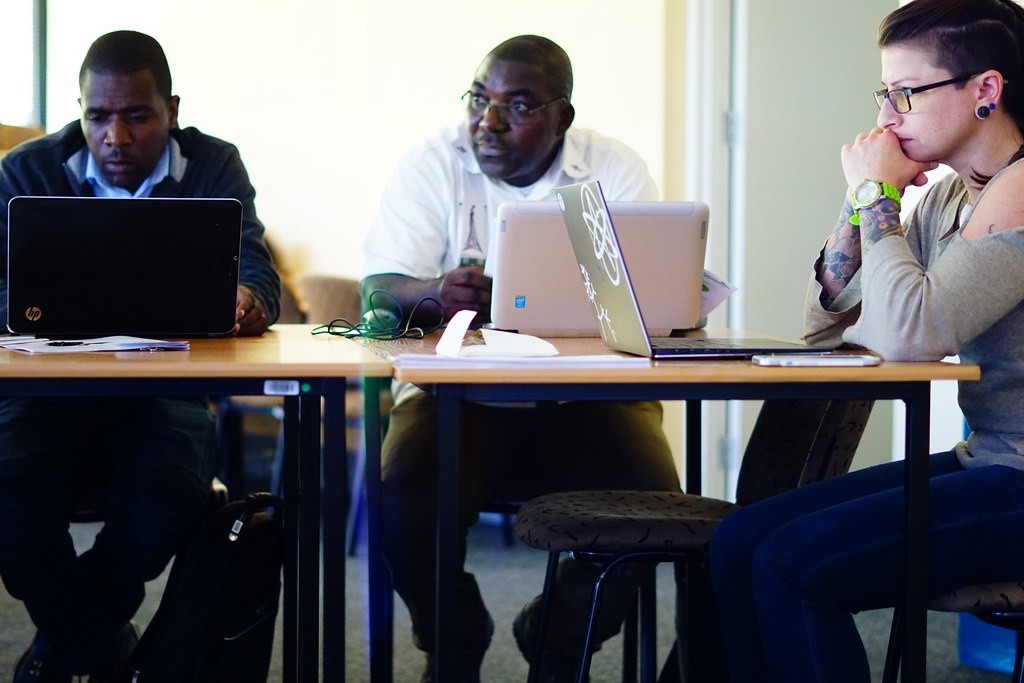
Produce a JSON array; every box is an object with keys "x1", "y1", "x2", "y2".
[
  {"x1": 64, "y1": 266, "x2": 660, "y2": 683},
  {"x1": 518, "y1": 343, "x2": 1024, "y2": 683}
]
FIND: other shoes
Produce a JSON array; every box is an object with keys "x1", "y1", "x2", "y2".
[
  {"x1": 89, "y1": 622, "x2": 140, "y2": 682},
  {"x1": 421, "y1": 618, "x2": 493, "y2": 683},
  {"x1": 512, "y1": 612, "x2": 600, "y2": 683},
  {"x1": 14, "y1": 635, "x2": 75, "y2": 682}
]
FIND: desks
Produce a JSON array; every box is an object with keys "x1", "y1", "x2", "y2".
[{"x1": 0, "y1": 323, "x2": 979, "y2": 683}]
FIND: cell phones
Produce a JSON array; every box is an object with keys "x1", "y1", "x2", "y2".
[{"x1": 752, "y1": 356, "x2": 880, "y2": 367}]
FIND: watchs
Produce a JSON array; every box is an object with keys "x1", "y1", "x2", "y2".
[{"x1": 849, "y1": 178, "x2": 902, "y2": 226}]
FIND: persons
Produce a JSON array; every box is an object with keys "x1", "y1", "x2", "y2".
[
  {"x1": 0, "y1": 30, "x2": 283, "y2": 683},
  {"x1": 358, "y1": 34, "x2": 685, "y2": 683},
  {"x1": 708, "y1": 0, "x2": 1024, "y2": 683}
]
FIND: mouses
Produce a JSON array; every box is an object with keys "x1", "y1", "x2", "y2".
[{"x1": 363, "y1": 308, "x2": 400, "y2": 335}]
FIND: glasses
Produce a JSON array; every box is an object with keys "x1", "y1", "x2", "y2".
[
  {"x1": 447, "y1": 92, "x2": 566, "y2": 124},
  {"x1": 872, "y1": 72, "x2": 985, "y2": 115}
]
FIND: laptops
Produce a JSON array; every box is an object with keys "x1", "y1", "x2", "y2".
[
  {"x1": 7, "y1": 196, "x2": 242, "y2": 340},
  {"x1": 483, "y1": 201, "x2": 709, "y2": 338},
  {"x1": 552, "y1": 179, "x2": 833, "y2": 363}
]
